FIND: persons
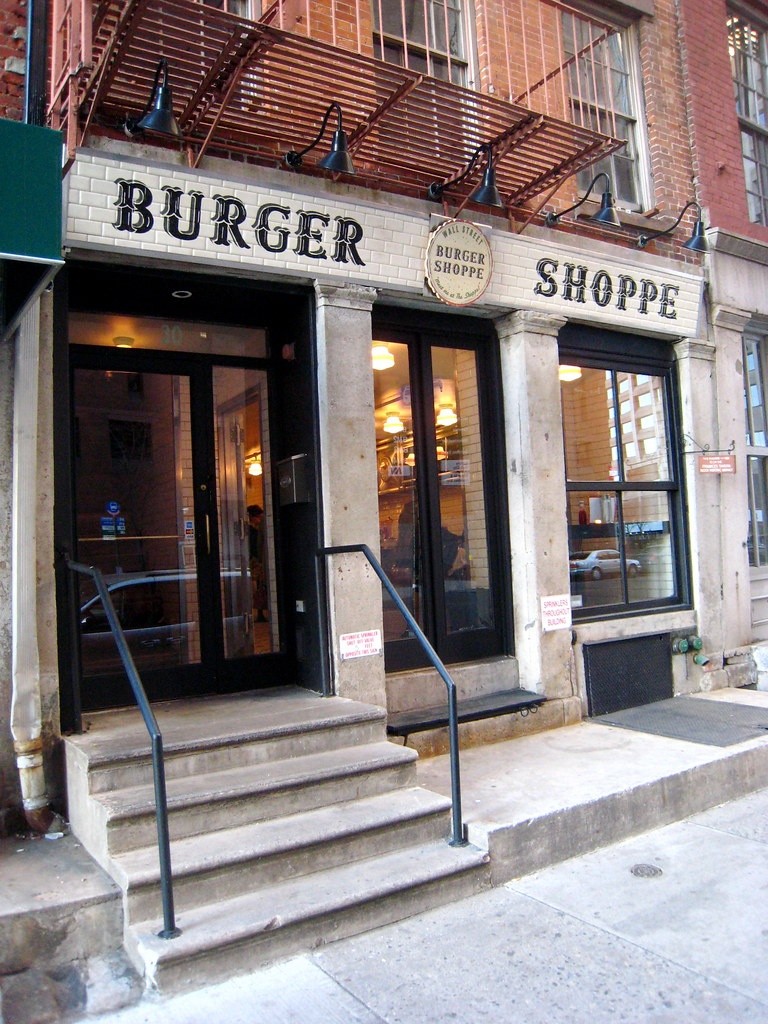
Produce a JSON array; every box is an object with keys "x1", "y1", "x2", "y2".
[
  {"x1": 578, "y1": 504, "x2": 589, "y2": 526},
  {"x1": 247, "y1": 505, "x2": 270, "y2": 623},
  {"x1": 395, "y1": 491, "x2": 418, "y2": 565}
]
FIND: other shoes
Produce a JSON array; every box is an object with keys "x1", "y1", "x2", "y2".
[{"x1": 256, "y1": 616, "x2": 268, "y2": 622}]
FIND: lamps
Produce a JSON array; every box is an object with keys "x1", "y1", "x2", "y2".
[
  {"x1": 249, "y1": 453, "x2": 262, "y2": 476},
  {"x1": 284, "y1": 104, "x2": 354, "y2": 174},
  {"x1": 428, "y1": 143, "x2": 504, "y2": 207},
  {"x1": 544, "y1": 172, "x2": 621, "y2": 228},
  {"x1": 636, "y1": 201, "x2": 710, "y2": 254},
  {"x1": 122, "y1": 58, "x2": 182, "y2": 141},
  {"x1": 371, "y1": 346, "x2": 395, "y2": 370},
  {"x1": 437, "y1": 408, "x2": 459, "y2": 425},
  {"x1": 383, "y1": 413, "x2": 404, "y2": 434},
  {"x1": 559, "y1": 364, "x2": 583, "y2": 381}
]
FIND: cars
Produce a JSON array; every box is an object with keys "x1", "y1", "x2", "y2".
[
  {"x1": 569, "y1": 548, "x2": 642, "y2": 582},
  {"x1": 379, "y1": 546, "x2": 414, "y2": 590},
  {"x1": 78, "y1": 566, "x2": 266, "y2": 695}
]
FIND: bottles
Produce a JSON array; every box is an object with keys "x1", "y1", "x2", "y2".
[
  {"x1": 578, "y1": 501, "x2": 589, "y2": 526},
  {"x1": 604, "y1": 495, "x2": 614, "y2": 523}
]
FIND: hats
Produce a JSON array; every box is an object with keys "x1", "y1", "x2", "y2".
[{"x1": 247, "y1": 505, "x2": 263, "y2": 516}]
